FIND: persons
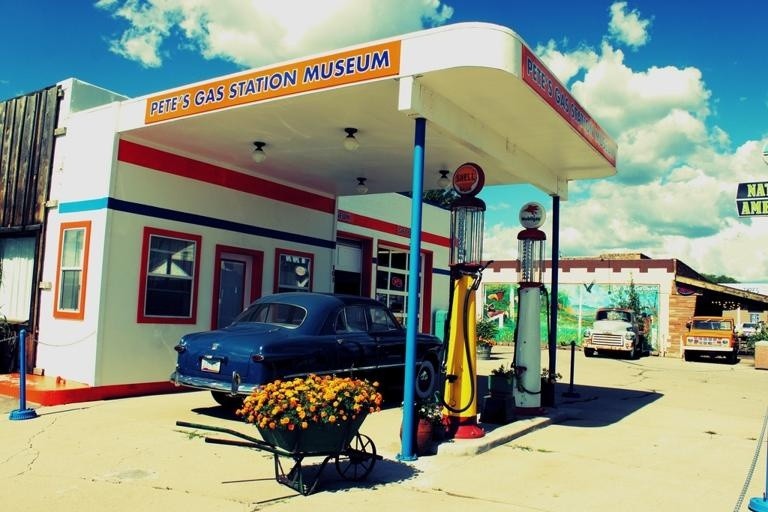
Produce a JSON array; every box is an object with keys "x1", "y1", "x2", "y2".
[{"x1": 637, "y1": 315, "x2": 651, "y2": 356}]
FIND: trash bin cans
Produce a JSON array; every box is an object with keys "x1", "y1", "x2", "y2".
[{"x1": 434, "y1": 310, "x2": 449, "y2": 343}]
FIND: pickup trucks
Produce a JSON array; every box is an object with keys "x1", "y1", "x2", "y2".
[{"x1": 682, "y1": 315, "x2": 740, "y2": 364}]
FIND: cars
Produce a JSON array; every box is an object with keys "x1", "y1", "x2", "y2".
[
  {"x1": 583, "y1": 307, "x2": 648, "y2": 360},
  {"x1": 168, "y1": 290, "x2": 444, "y2": 408}
]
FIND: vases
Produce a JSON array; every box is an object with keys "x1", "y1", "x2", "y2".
[
  {"x1": 489, "y1": 375, "x2": 513, "y2": 396},
  {"x1": 476, "y1": 344, "x2": 492, "y2": 360},
  {"x1": 401, "y1": 416, "x2": 434, "y2": 455}
]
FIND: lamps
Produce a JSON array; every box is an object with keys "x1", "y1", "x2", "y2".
[
  {"x1": 355, "y1": 176, "x2": 370, "y2": 195},
  {"x1": 251, "y1": 141, "x2": 268, "y2": 165},
  {"x1": 342, "y1": 127, "x2": 360, "y2": 153},
  {"x1": 437, "y1": 170, "x2": 452, "y2": 188}
]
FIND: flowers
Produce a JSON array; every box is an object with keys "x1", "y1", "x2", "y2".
[
  {"x1": 399, "y1": 399, "x2": 444, "y2": 423},
  {"x1": 473, "y1": 319, "x2": 500, "y2": 348},
  {"x1": 235, "y1": 372, "x2": 381, "y2": 432},
  {"x1": 541, "y1": 367, "x2": 562, "y2": 385},
  {"x1": 491, "y1": 365, "x2": 513, "y2": 378}
]
